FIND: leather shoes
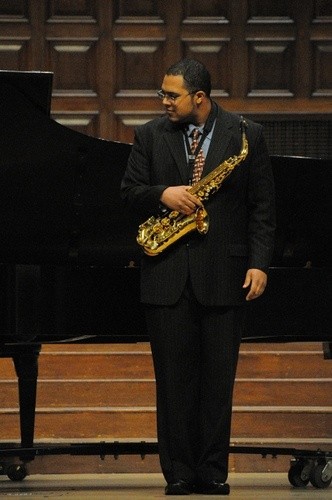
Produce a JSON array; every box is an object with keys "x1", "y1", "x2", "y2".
[
  {"x1": 193, "y1": 479, "x2": 231, "y2": 496},
  {"x1": 165, "y1": 479, "x2": 193, "y2": 494}
]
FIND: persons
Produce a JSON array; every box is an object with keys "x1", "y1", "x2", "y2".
[{"x1": 119, "y1": 59, "x2": 277, "y2": 495}]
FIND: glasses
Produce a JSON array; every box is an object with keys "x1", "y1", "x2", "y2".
[{"x1": 156, "y1": 89, "x2": 199, "y2": 103}]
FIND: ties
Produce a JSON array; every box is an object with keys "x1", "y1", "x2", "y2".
[{"x1": 189, "y1": 128, "x2": 205, "y2": 186}]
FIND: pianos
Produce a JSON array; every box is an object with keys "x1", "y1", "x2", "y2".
[{"x1": 0, "y1": 71, "x2": 331, "y2": 485}]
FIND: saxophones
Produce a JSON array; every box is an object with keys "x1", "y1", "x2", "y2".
[{"x1": 135, "y1": 115, "x2": 250, "y2": 257}]
